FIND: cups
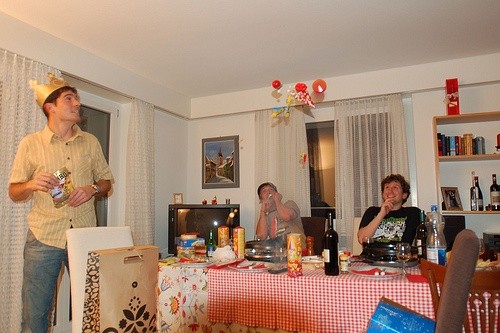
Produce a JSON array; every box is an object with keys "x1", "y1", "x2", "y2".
[{"x1": 226, "y1": 199, "x2": 230, "y2": 205}]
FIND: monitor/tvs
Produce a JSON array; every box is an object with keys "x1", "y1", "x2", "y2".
[{"x1": 168, "y1": 203, "x2": 241, "y2": 253}]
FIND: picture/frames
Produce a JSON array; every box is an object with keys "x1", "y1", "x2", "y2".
[{"x1": 202, "y1": 135, "x2": 240, "y2": 189}]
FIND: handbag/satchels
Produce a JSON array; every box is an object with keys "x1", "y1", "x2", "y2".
[{"x1": 81, "y1": 245, "x2": 159, "y2": 333}]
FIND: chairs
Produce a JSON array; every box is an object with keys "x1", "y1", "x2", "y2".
[
  {"x1": 420, "y1": 258, "x2": 499, "y2": 333},
  {"x1": 300, "y1": 216, "x2": 327, "y2": 254}
]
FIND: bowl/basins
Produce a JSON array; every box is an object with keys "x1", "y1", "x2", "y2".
[{"x1": 228, "y1": 260, "x2": 274, "y2": 272}]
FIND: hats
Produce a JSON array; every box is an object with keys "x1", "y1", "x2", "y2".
[{"x1": 257, "y1": 182, "x2": 277, "y2": 197}]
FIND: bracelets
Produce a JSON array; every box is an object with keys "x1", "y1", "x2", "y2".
[{"x1": 91, "y1": 184, "x2": 99, "y2": 192}]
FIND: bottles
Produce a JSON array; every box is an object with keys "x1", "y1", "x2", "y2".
[
  {"x1": 306, "y1": 235, "x2": 314, "y2": 255},
  {"x1": 469, "y1": 171, "x2": 483, "y2": 211},
  {"x1": 338, "y1": 251, "x2": 351, "y2": 275},
  {"x1": 490, "y1": 174, "x2": 500, "y2": 211},
  {"x1": 207, "y1": 230, "x2": 216, "y2": 257},
  {"x1": 416, "y1": 210, "x2": 427, "y2": 263},
  {"x1": 322, "y1": 212, "x2": 339, "y2": 276},
  {"x1": 424, "y1": 205, "x2": 448, "y2": 267}
]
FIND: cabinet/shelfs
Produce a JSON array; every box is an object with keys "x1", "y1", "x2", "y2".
[{"x1": 432, "y1": 110, "x2": 500, "y2": 246}]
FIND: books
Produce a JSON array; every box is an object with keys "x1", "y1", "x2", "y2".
[{"x1": 437, "y1": 132, "x2": 485, "y2": 156}]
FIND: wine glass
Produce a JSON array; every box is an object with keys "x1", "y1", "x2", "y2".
[{"x1": 394, "y1": 243, "x2": 411, "y2": 279}]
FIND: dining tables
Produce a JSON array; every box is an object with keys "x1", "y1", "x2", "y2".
[{"x1": 153, "y1": 254, "x2": 500, "y2": 333}]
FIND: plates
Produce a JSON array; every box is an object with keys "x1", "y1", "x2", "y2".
[
  {"x1": 177, "y1": 261, "x2": 213, "y2": 267},
  {"x1": 301, "y1": 254, "x2": 324, "y2": 264},
  {"x1": 348, "y1": 264, "x2": 403, "y2": 278}
]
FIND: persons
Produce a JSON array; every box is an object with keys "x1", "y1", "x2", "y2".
[
  {"x1": 256, "y1": 182, "x2": 307, "y2": 248},
  {"x1": 8, "y1": 86, "x2": 114, "y2": 333},
  {"x1": 357, "y1": 173, "x2": 421, "y2": 258}
]
